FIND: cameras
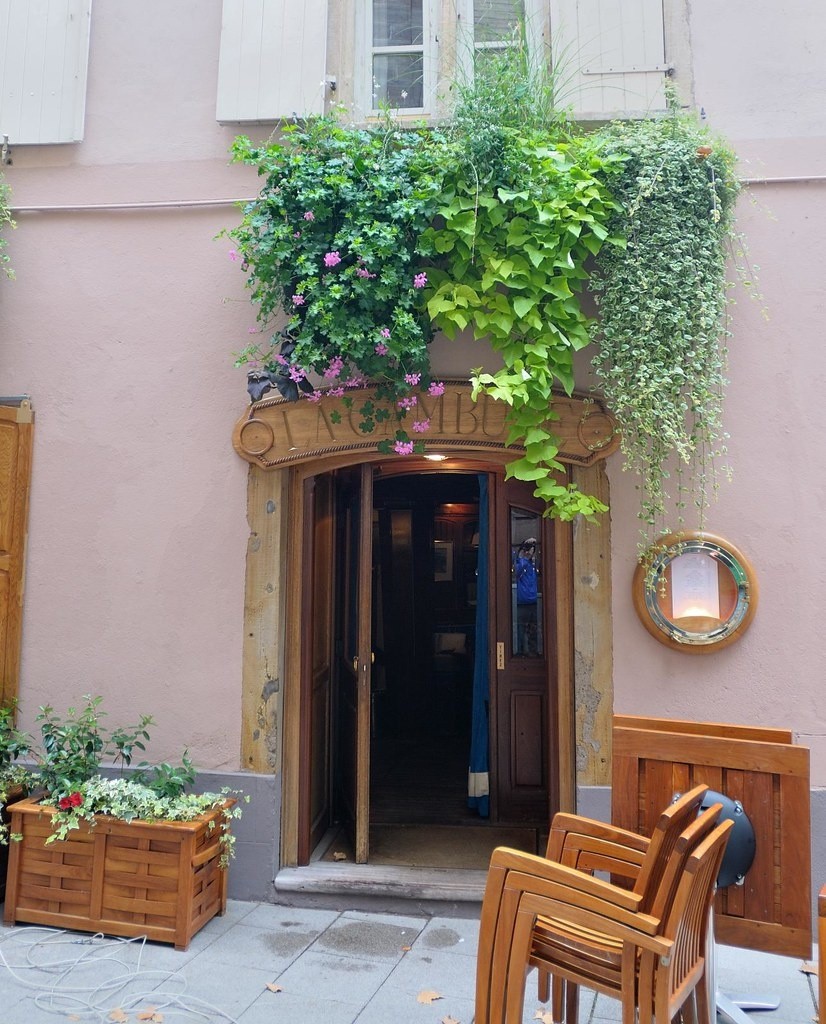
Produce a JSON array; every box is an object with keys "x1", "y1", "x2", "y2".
[{"x1": 521, "y1": 541, "x2": 536, "y2": 553}]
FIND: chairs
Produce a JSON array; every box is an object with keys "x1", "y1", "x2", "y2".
[{"x1": 473, "y1": 783, "x2": 733, "y2": 1024}]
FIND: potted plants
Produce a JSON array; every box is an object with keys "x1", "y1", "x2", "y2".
[{"x1": 0, "y1": 694, "x2": 250, "y2": 952}]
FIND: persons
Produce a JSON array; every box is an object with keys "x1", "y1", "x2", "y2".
[{"x1": 516, "y1": 539, "x2": 543, "y2": 657}]
